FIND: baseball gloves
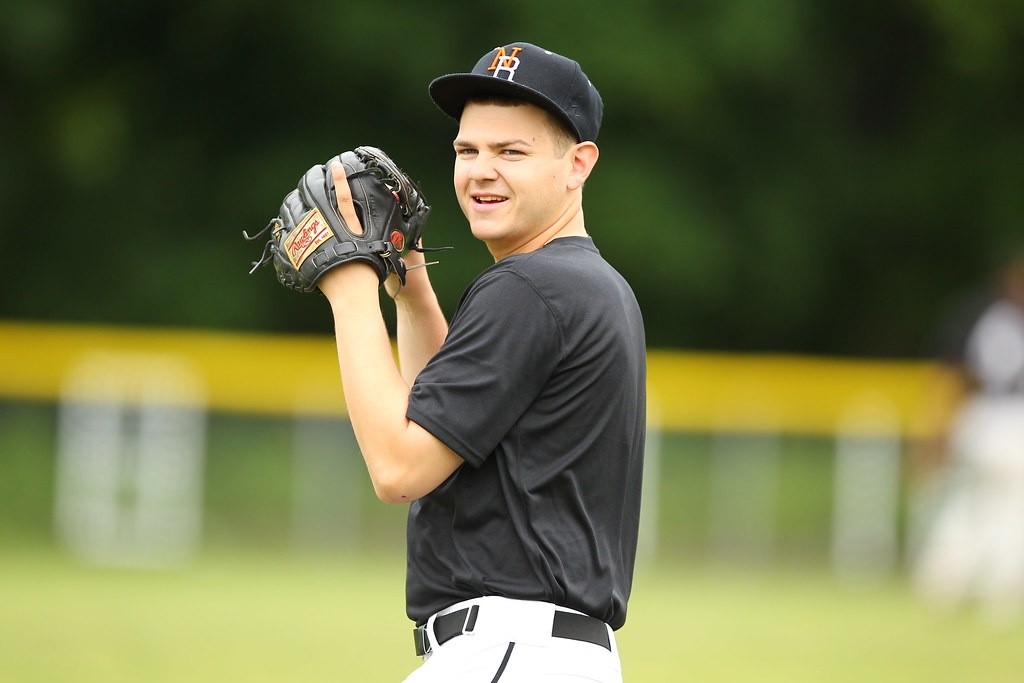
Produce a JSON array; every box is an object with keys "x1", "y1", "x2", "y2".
[{"x1": 240, "y1": 142, "x2": 455, "y2": 294}]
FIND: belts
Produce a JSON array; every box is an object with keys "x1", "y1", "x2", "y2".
[{"x1": 412, "y1": 603, "x2": 617, "y2": 655}]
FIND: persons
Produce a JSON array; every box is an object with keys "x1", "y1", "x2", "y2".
[
  {"x1": 917, "y1": 254, "x2": 1024, "y2": 601},
  {"x1": 274, "y1": 41, "x2": 646, "y2": 683}
]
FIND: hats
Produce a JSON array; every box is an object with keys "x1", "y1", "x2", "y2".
[{"x1": 427, "y1": 40, "x2": 603, "y2": 146}]
